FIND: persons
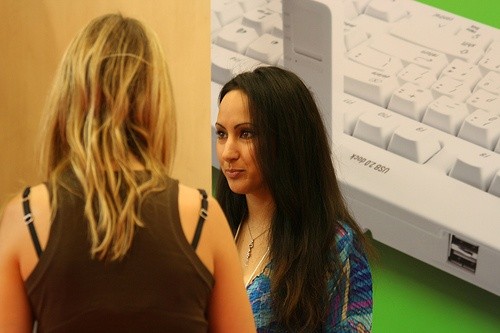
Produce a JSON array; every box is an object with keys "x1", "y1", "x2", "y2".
[
  {"x1": 216, "y1": 67, "x2": 372, "y2": 333},
  {"x1": 0, "y1": 13, "x2": 257, "y2": 333}
]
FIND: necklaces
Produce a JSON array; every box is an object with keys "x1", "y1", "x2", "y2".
[{"x1": 245, "y1": 219, "x2": 272, "y2": 261}]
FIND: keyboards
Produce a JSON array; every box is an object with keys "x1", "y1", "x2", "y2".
[{"x1": 211, "y1": 0, "x2": 500, "y2": 296}]
